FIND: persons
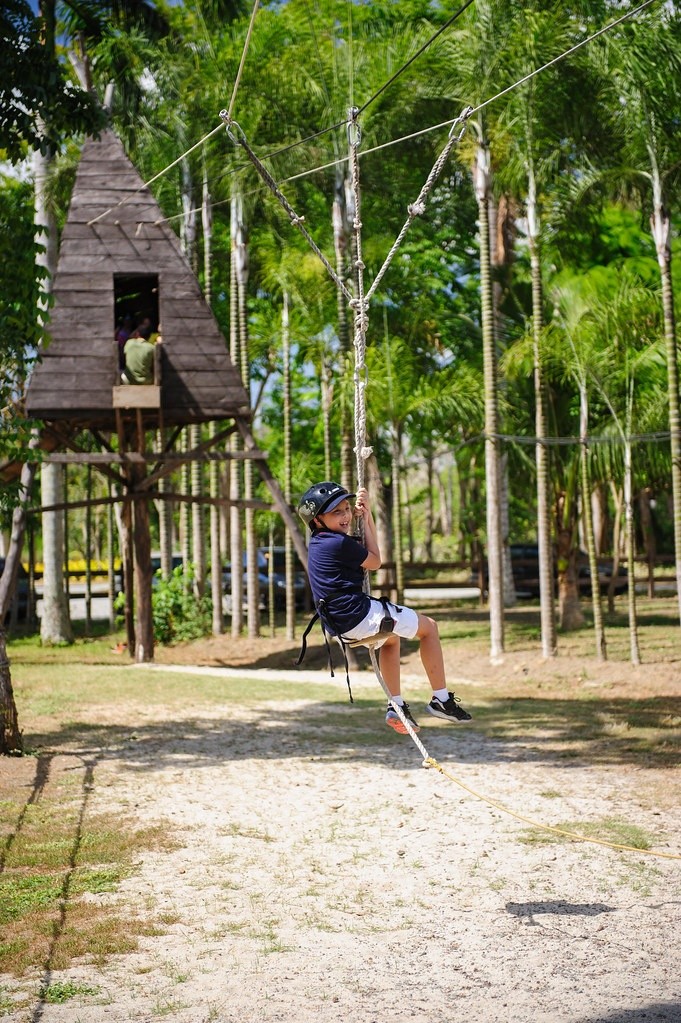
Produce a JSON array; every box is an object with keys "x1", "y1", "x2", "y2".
[
  {"x1": 296, "y1": 481, "x2": 471, "y2": 734},
  {"x1": 114, "y1": 313, "x2": 164, "y2": 386}
]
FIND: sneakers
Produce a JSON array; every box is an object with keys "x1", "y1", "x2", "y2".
[
  {"x1": 386, "y1": 701, "x2": 420, "y2": 736},
  {"x1": 428, "y1": 691, "x2": 473, "y2": 724}
]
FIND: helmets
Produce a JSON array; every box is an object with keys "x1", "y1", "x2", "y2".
[{"x1": 297, "y1": 481, "x2": 348, "y2": 532}]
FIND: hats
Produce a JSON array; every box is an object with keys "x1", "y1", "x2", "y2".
[{"x1": 316, "y1": 491, "x2": 356, "y2": 514}]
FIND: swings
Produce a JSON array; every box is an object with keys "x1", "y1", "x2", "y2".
[{"x1": 216, "y1": 102, "x2": 681, "y2": 861}]
